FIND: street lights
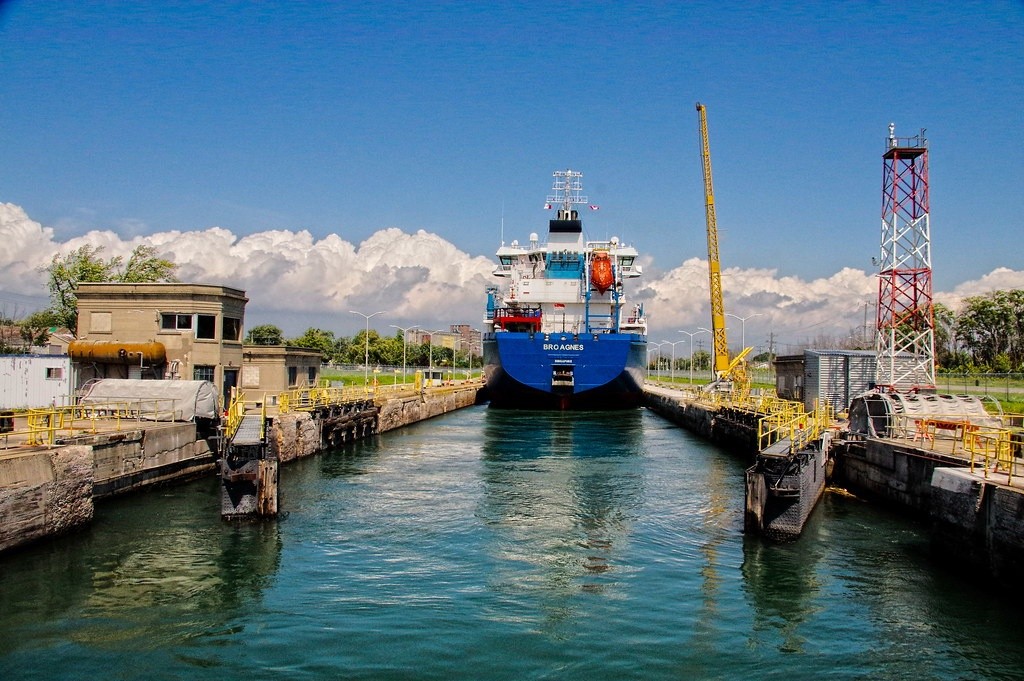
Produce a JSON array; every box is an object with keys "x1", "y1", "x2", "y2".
[
  {"x1": 647, "y1": 342, "x2": 666, "y2": 381},
  {"x1": 726, "y1": 313, "x2": 763, "y2": 351},
  {"x1": 697, "y1": 327, "x2": 730, "y2": 381},
  {"x1": 446, "y1": 337, "x2": 481, "y2": 380},
  {"x1": 349, "y1": 311, "x2": 385, "y2": 385},
  {"x1": 418, "y1": 329, "x2": 443, "y2": 379},
  {"x1": 662, "y1": 340, "x2": 685, "y2": 385},
  {"x1": 646, "y1": 348, "x2": 658, "y2": 380},
  {"x1": 678, "y1": 330, "x2": 705, "y2": 385},
  {"x1": 388, "y1": 325, "x2": 419, "y2": 384}
]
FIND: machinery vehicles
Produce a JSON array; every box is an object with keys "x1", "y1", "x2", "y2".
[{"x1": 696, "y1": 100, "x2": 754, "y2": 396}]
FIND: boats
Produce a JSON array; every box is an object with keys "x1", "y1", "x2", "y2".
[{"x1": 482, "y1": 168, "x2": 649, "y2": 411}]
